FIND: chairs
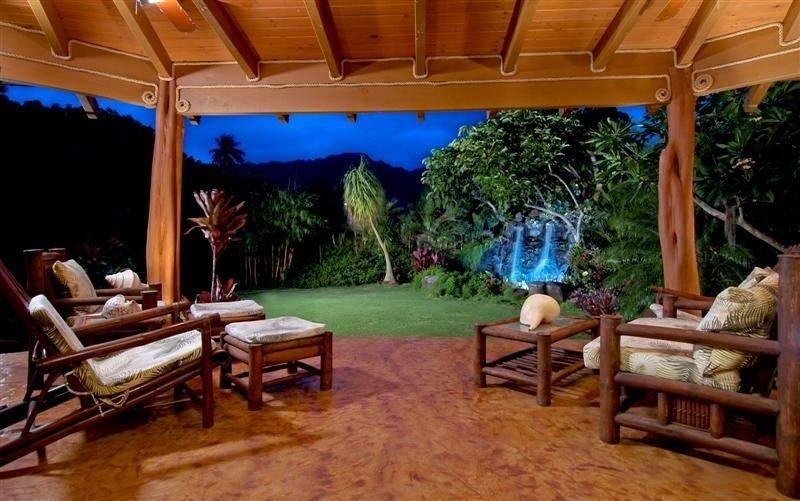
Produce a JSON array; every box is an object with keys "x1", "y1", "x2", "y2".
[
  {"x1": 584, "y1": 247, "x2": 799, "y2": 500},
  {"x1": 1, "y1": 242, "x2": 221, "y2": 466}
]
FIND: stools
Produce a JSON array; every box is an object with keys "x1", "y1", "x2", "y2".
[{"x1": 184, "y1": 301, "x2": 334, "y2": 411}]
